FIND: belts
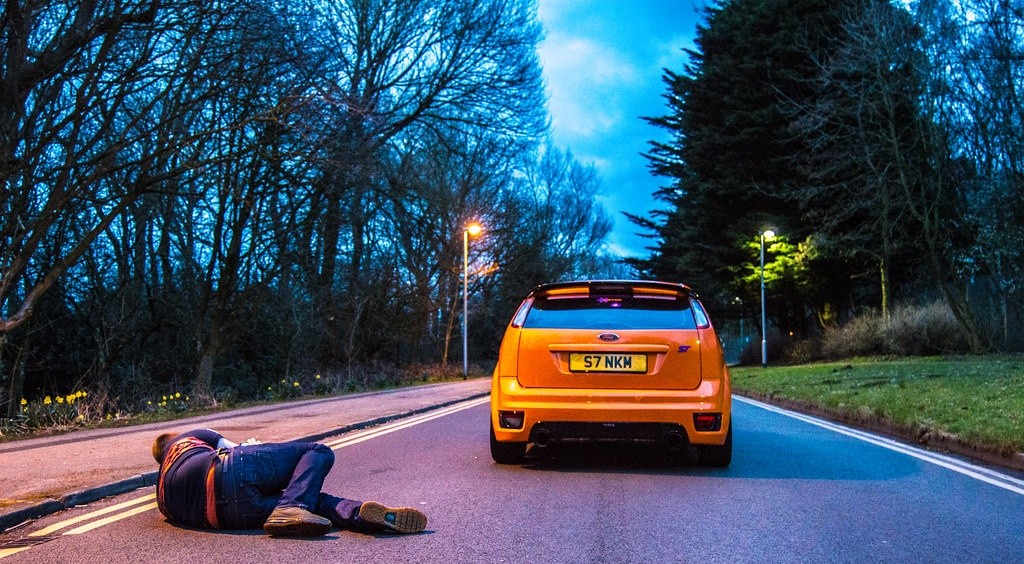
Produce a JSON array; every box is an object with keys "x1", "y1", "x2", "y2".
[{"x1": 213, "y1": 449, "x2": 227, "y2": 530}]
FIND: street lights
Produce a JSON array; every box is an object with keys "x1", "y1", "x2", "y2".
[
  {"x1": 460, "y1": 223, "x2": 481, "y2": 380},
  {"x1": 758, "y1": 229, "x2": 776, "y2": 367}
]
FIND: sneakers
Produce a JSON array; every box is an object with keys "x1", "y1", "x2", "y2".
[
  {"x1": 263, "y1": 506, "x2": 333, "y2": 538},
  {"x1": 359, "y1": 501, "x2": 429, "y2": 533}
]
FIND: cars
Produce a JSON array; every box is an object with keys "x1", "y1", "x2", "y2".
[{"x1": 486, "y1": 280, "x2": 733, "y2": 464}]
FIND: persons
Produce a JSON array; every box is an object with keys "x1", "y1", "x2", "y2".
[{"x1": 152, "y1": 426, "x2": 430, "y2": 534}]
